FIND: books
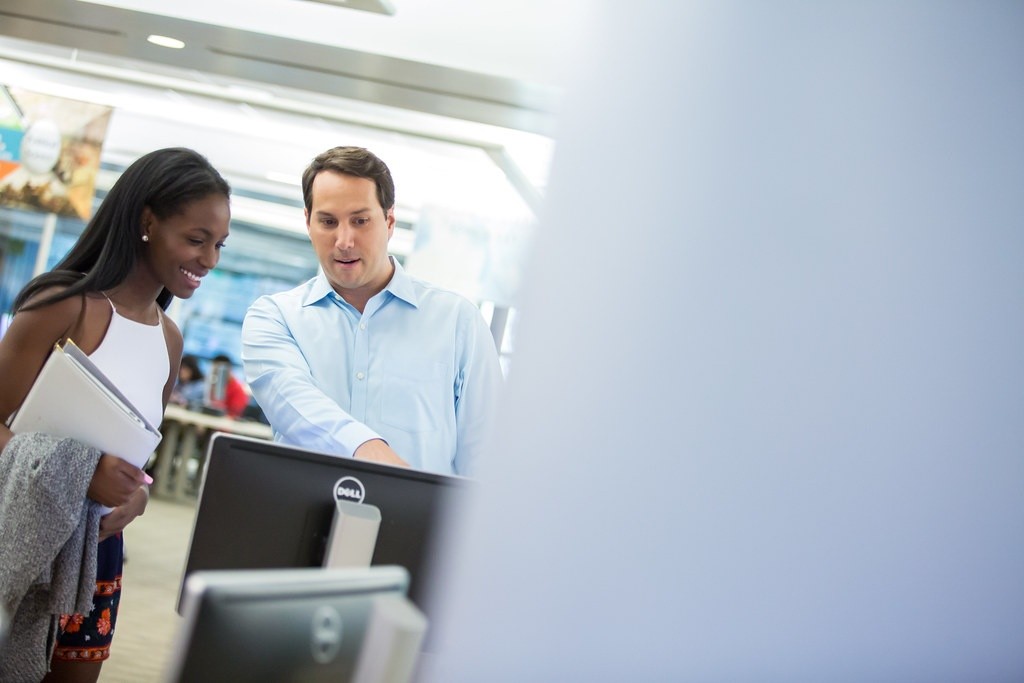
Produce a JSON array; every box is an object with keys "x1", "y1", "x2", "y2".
[{"x1": 10, "y1": 337, "x2": 163, "y2": 518}]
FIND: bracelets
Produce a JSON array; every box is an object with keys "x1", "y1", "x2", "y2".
[{"x1": 138, "y1": 485, "x2": 149, "y2": 517}]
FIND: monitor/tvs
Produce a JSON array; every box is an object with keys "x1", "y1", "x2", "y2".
[{"x1": 165, "y1": 431, "x2": 471, "y2": 683}]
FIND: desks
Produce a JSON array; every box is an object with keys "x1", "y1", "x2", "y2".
[{"x1": 150, "y1": 405, "x2": 273, "y2": 501}]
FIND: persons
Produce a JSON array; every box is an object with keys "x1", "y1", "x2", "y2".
[
  {"x1": 149, "y1": 354, "x2": 272, "y2": 488},
  {"x1": 240, "y1": 146, "x2": 505, "y2": 481},
  {"x1": 0, "y1": 147, "x2": 232, "y2": 683}
]
